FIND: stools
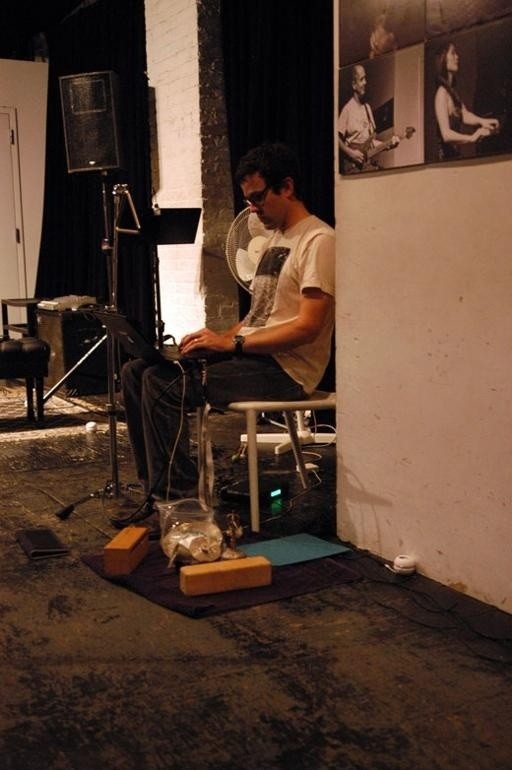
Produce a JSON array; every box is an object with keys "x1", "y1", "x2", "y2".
[
  {"x1": 197, "y1": 392, "x2": 338, "y2": 535},
  {"x1": 2, "y1": 335, "x2": 51, "y2": 418}
]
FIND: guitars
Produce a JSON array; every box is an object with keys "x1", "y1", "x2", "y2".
[{"x1": 340, "y1": 127, "x2": 416, "y2": 173}]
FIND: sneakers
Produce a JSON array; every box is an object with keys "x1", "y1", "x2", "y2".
[{"x1": 111, "y1": 502, "x2": 162, "y2": 541}]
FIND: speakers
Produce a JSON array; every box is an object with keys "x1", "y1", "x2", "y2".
[
  {"x1": 57, "y1": 69, "x2": 127, "y2": 174},
  {"x1": 34, "y1": 309, "x2": 122, "y2": 398}
]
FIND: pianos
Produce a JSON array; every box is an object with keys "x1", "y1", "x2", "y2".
[{"x1": 449, "y1": 108, "x2": 512, "y2": 160}]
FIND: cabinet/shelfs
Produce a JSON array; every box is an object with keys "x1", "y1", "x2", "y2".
[{"x1": 5, "y1": 296, "x2": 109, "y2": 399}]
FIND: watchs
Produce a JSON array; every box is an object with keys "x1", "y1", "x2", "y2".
[{"x1": 232, "y1": 334, "x2": 245, "y2": 357}]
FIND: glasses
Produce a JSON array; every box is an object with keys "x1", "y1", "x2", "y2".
[{"x1": 245, "y1": 186, "x2": 270, "y2": 204}]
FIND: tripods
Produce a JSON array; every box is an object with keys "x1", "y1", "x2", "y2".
[{"x1": 54, "y1": 182, "x2": 166, "y2": 519}]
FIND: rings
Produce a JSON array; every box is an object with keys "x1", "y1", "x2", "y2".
[{"x1": 194, "y1": 338, "x2": 198, "y2": 343}]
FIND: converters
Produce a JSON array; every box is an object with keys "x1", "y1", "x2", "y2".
[
  {"x1": 297, "y1": 463, "x2": 319, "y2": 472},
  {"x1": 86, "y1": 421, "x2": 97, "y2": 432}
]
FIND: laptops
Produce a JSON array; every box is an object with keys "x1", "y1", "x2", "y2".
[{"x1": 91, "y1": 311, "x2": 233, "y2": 366}]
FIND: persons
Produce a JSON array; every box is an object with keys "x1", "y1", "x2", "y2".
[
  {"x1": 366, "y1": 1, "x2": 426, "y2": 59},
  {"x1": 339, "y1": 64, "x2": 401, "y2": 174},
  {"x1": 110, "y1": 144, "x2": 335, "y2": 542},
  {"x1": 428, "y1": 40, "x2": 499, "y2": 162}
]
